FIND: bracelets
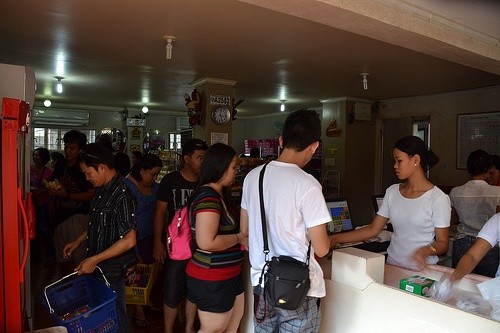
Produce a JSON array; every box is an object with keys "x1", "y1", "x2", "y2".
[{"x1": 428, "y1": 244, "x2": 437, "y2": 256}]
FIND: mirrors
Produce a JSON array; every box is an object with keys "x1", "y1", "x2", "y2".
[{"x1": 100, "y1": 127, "x2": 127, "y2": 155}]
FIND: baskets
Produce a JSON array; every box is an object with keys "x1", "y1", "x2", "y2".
[
  {"x1": 40, "y1": 265, "x2": 120, "y2": 333},
  {"x1": 124, "y1": 264, "x2": 155, "y2": 305}
]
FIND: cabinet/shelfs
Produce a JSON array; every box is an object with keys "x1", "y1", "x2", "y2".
[{"x1": 225, "y1": 158, "x2": 268, "y2": 215}]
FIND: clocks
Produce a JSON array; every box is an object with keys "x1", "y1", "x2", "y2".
[{"x1": 211, "y1": 105, "x2": 231, "y2": 127}]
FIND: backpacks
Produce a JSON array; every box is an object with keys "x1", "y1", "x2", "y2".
[{"x1": 163, "y1": 189, "x2": 193, "y2": 262}]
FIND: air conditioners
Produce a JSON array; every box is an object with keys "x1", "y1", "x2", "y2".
[{"x1": 31, "y1": 107, "x2": 90, "y2": 128}]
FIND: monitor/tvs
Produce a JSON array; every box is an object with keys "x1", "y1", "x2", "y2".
[
  {"x1": 326, "y1": 198, "x2": 354, "y2": 237},
  {"x1": 372, "y1": 195, "x2": 391, "y2": 223}
]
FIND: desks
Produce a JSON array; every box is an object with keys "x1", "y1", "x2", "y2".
[{"x1": 238, "y1": 242, "x2": 500, "y2": 333}]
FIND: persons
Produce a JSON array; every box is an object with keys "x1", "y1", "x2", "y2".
[
  {"x1": 62, "y1": 143, "x2": 139, "y2": 333},
  {"x1": 329, "y1": 135, "x2": 452, "y2": 270},
  {"x1": 249, "y1": 146, "x2": 262, "y2": 158},
  {"x1": 448, "y1": 149, "x2": 500, "y2": 282},
  {"x1": 238, "y1": 110, "x2": 333, "y2": 333},
  {"x1": 28, "y1": 131, "x2": 165, "y2": 327},
  {"x1": 153, "y1": 138, "x2": 209, "y2": 333},
  {"x1": 186, "y1": 143, "x2": 248, "y2": 333}
]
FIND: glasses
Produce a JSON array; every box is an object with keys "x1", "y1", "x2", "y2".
[{"x1": 77, "y1": 149, "x2": 98, "y2": 161}]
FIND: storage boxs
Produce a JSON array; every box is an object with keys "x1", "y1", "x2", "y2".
[{"x1": 400, "y1": 275, "x2": 436, "y2": 295}]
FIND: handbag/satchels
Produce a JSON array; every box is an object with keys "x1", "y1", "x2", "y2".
[{"x1": 263, "y1": 254, "x2": 311, "y2": 310}]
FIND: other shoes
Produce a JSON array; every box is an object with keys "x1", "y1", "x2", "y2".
[{"x1": 133, "y1": 315, "x2": 151, "y2": 329}]
